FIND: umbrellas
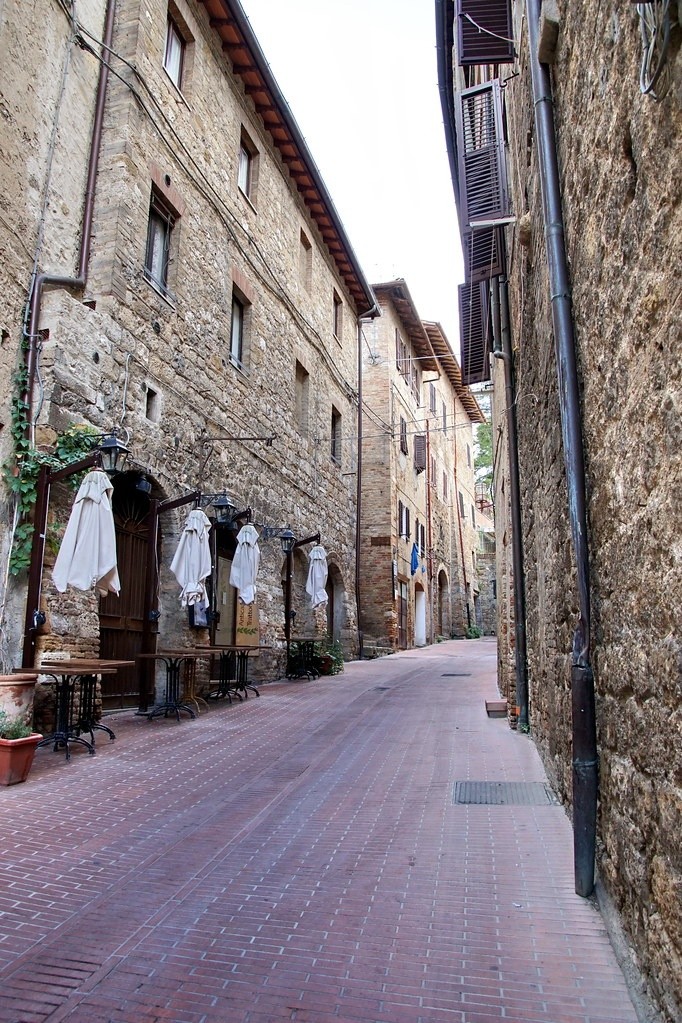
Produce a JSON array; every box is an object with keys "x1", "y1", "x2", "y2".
[
  {"x1": 22, "y1": 450, "x2": 121, "y2": 668},
  {"x1": 211, "y1": 504, "x2": 260, "y2": 681},
  {"x1": 286, "y1": 531, "x2": 329, "y2": 679},
  {"x1": 134, "y1": 487, "x2": 212, "y2": 716}
]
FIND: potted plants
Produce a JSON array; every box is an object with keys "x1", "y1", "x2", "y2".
[{"x1": 0, "y1": 711, "x2": 43, "y2": 787}]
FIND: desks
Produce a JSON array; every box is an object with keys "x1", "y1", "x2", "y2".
[
  {"x1": 277, "y1": 637, "x2": 316, "y2": 682},
  {"x1": 224, "y1": 641, "x2": 273, "y2": 699},
  {"x1": 136, "y1": 653, "x2": 198, "y2": 721},
  {"x1": 10, "y1": 666, "x2": 116, "y2": 760},
  {"x1": 42, "y1": 658, "x2": 137, "y2": 749},
  {"x1": 158, "y1": 646, "x2": 221, "y2": 716},
  {"x1": 197, "y1": 644, "x2": 246, "y2": 703},
  {"x1": 299, "y1": 636, "x2": 324, "y2": 682}
]
